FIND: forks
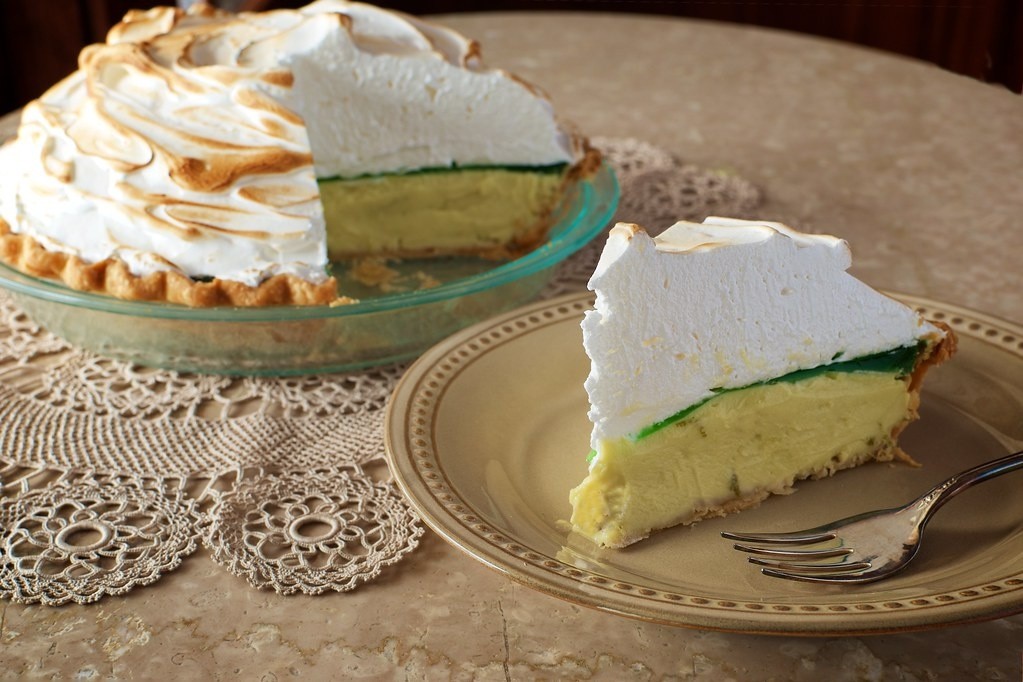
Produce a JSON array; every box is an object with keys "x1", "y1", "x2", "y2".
[{"x1": 720, "y1": 449, "x2": 1023, "y2": 582}]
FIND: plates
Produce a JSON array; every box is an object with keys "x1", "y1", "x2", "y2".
[
  {"x1": 0, "y1": 99, "x2": 623, "y2": 378},
  {"x1": 383, "y1": 286, "x2": 1023, "y2": 637}
]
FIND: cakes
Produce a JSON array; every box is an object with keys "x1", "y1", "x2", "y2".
[
  {"x1": 567, "y1": 213, "x2": 958, "y2": 550},
  {"x1": 0, "y1": 0, "x2": 603, "y2": 362}
]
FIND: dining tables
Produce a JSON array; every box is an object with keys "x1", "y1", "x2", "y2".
[{"x1": 0, "y1": 15, "x2": 1023, "y2": 682}]
